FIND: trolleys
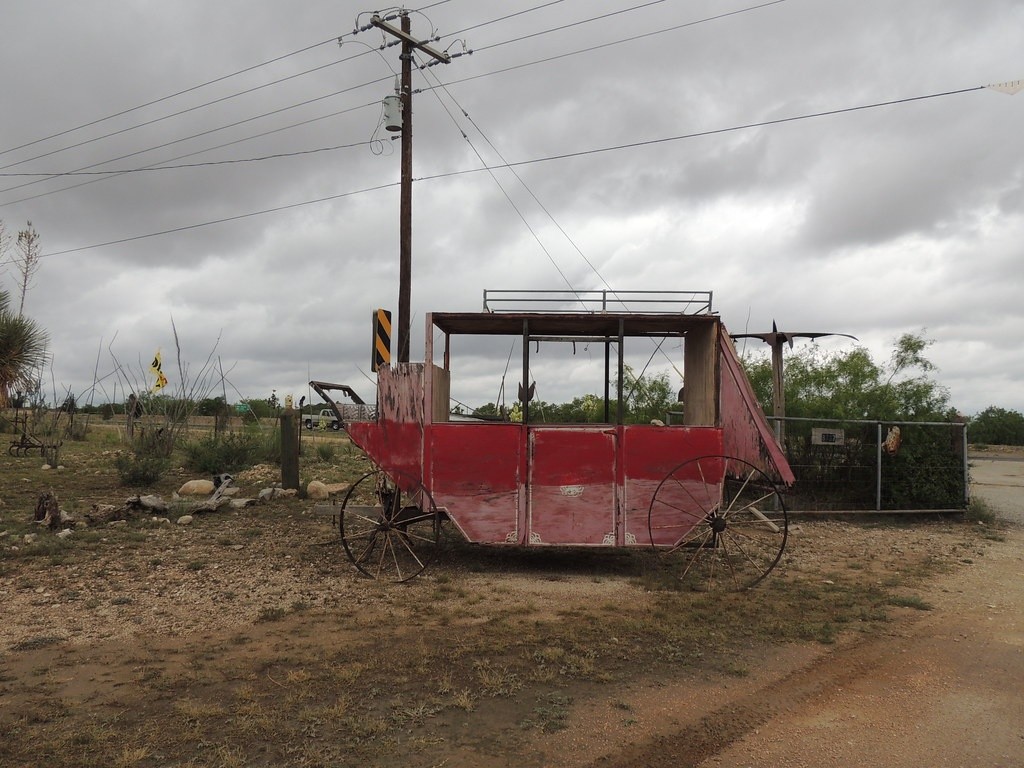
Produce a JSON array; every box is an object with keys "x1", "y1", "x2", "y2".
[{"x1": 307, "y1": 289, "x2": 794, "y2": 594}]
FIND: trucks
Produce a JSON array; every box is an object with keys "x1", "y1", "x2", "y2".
[{"x1": 301, "y1": 409, "x2": 343, "y2": 430}]
